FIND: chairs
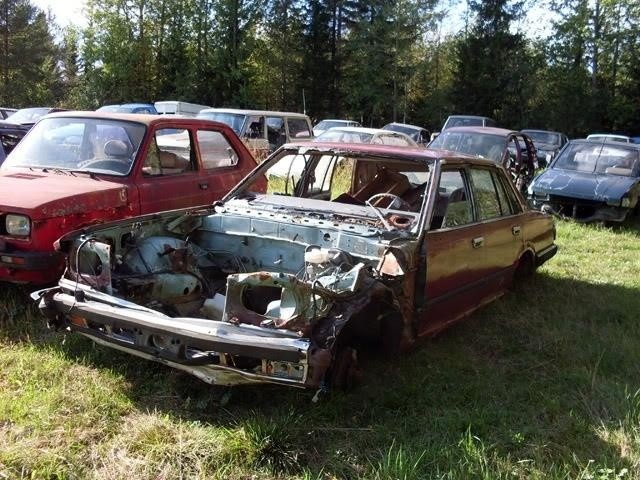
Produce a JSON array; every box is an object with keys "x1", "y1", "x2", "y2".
[
  {"x1": 139, "y1": 153, "x2": 183, "y2": 177},
  {"x1": 104, "y1": 140, "x2": 131, "y2": 164}
]
